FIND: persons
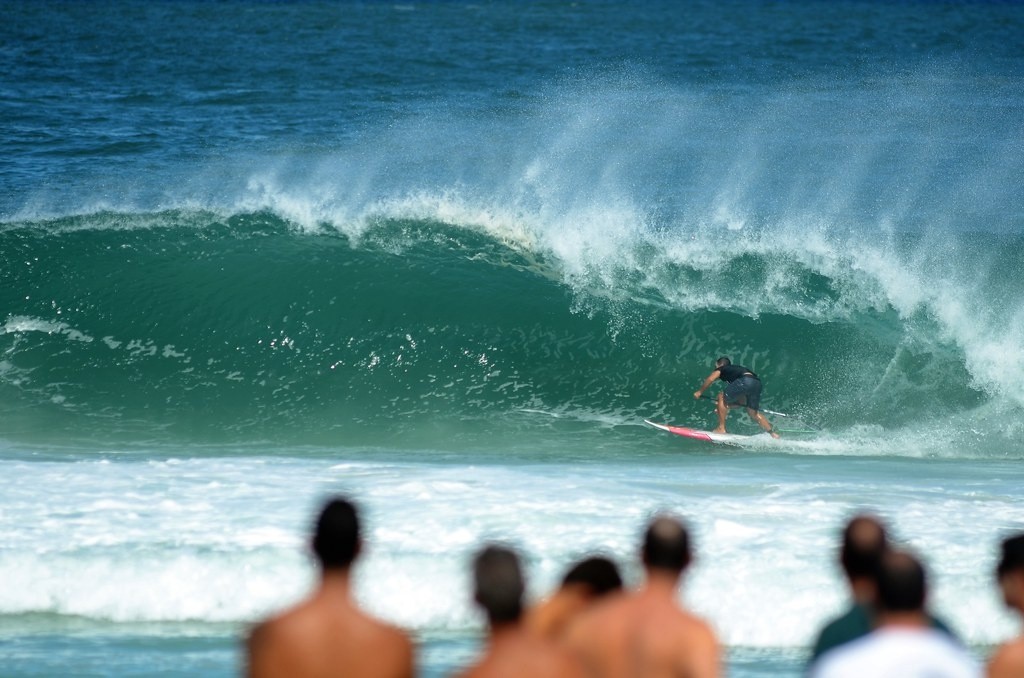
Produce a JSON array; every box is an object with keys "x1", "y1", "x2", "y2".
[
  {"x1": 247, "y1": 499, "x2": 414, "y2": 678},
  {"x1": 442, "y1": 517, "x2": 727, "y2": 678},
  {"x1": 809, "y1": 515, "x2": 1024, "y2": 678},
  {"x1": 694, "y1": 358, "x2": 780, "y2": 438}
]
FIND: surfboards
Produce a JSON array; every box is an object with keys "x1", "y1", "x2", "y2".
[{"x1": 643, "y1": 418, "x2": 751, "y2": 442}]
